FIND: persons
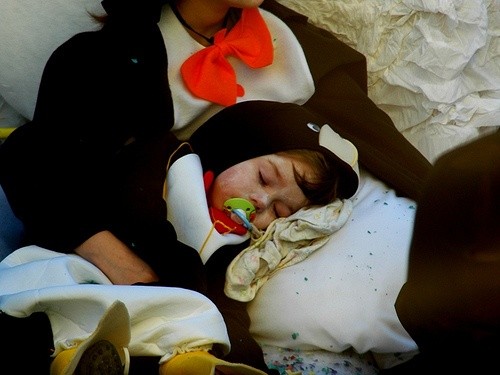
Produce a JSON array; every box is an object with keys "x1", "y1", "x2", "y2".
[{"x1": 0, "y1": 0, "x2": 437, "y2": 375}]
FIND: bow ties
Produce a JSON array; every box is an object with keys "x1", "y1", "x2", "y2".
[{"x1": 181, "y1": 5, "x2": 274, "y2": 106}]
